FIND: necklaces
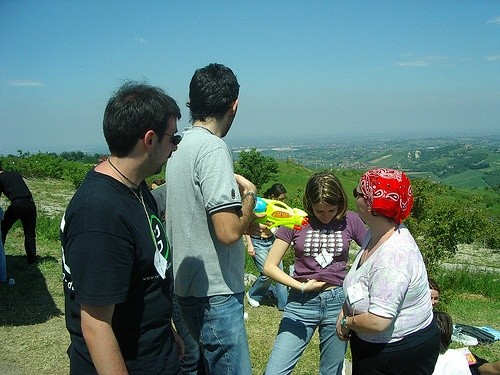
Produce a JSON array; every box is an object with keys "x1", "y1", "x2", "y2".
[
  {"x1": 368, "y1": 226, "x2": 395, "y2": 252},
  {"x1": 107, "y1": 159, "x2": 139, "y2": 188},
  {"x1": 193, "y1": 125, "x2": 213, "y2": 134}
]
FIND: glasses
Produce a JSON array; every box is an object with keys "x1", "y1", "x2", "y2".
[
  {"x1": 140, "y1": 130, "x2": 182, "y2": 145},
  {"x1": 353, "y1": 188, "x2": 363, "y2": 198}
]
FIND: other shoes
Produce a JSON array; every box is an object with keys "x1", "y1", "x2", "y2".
[{"x1": 27, "y1": 258, "x2": 37, "y2": 264}]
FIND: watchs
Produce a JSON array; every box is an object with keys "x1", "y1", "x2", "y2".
[{"x1": 341, "y1": 314, "x2": 350, "y2": 337}]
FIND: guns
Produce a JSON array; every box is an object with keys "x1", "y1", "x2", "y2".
[{"x1": 254, "y1": 196, "x2": 308, "y2": 231}]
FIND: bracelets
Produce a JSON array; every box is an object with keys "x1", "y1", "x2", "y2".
[
  {"x1": 242, "y1": 192, "x2": 256, "y2": 208},
  {"x1": 300, "y1": 281, "x2": 305, "y2": 294}
]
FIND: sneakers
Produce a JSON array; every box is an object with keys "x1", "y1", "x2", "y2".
[{"x1": 246, "y1": 291, "x2": 260, "y2": 307}]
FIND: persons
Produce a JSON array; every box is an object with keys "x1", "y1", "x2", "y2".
[
  {"x1": 151, "y1": 65, "x2": 500, "y2": 375},
  {"x1": 58, "y1": 80, "x2": 185, "y2": 375},
  {"x1": 0, "y1": 167, "x2": 37, "y2": 283}
]
